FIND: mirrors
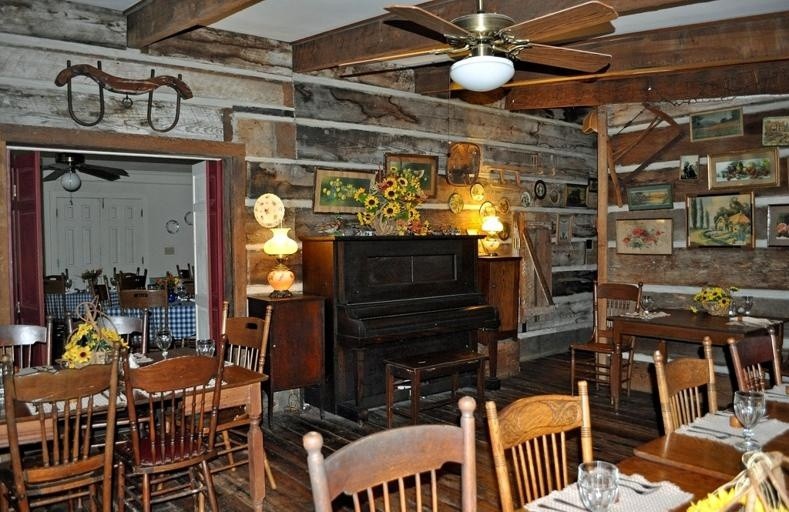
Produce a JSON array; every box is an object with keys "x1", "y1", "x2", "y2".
[{"x1": 446, "y1": 142, "x2": 480, "y2": 187}]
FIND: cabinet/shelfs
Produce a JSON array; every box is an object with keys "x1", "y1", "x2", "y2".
[
  {"x1": 250, "y1": 295, "x2": 333, "y2": 429},
  {"x1": 476, "y1": 258, "x2": 521, "y2": 378}
]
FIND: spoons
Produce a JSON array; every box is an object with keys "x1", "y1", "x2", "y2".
[
  {"x1": 620, "y1": 476, "x2": 663, "y2": 489},
  {"x1": 688, "y1": 430, "x2": 731, "y2": 439}
]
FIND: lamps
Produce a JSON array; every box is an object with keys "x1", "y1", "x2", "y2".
[
  {"x1": 481, "y1": 214, "x2": 505, "y2": 256},
  {"x1": 61, "y1": 169, "x2": 81, "y2": 191},
  {"x1": 448, "y1": 44, "x2": 515, "y2": 93},
  {"x1": 254, "y1": 192, "x2": 298, "y2": 299}
]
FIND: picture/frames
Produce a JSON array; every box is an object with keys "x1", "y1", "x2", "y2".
[
  {"x1": 564, "y1": 183, "x2": 589, "y2": 207},
  {"x1": 615, "y1": 106, "x2": 789, "y2": 255},
  {"x1": 556, "y1": 212, "x2": 574, "y2": 244},
  {"x1": 313, "y1": 152, "x2": 439, "y2": 214}
]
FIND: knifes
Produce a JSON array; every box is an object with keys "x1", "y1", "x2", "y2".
[{"x1": 691, "y1": 425, "x2": 759, "y2": 442}]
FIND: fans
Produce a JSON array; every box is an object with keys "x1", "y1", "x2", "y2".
[
  {"x1": 42, "y1": 153, "x2": 129, "y2": 182},
  {"x1": 377, "y1": 0, "x2": 619, "y2": 74}
]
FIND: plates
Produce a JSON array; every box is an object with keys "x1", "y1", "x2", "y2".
[
  {"x1": 254, "y1": 193, "x2": 285, "y2": 229},
  {"x1": 449, "y1": 179, "x2": 561, "y2": 241}
]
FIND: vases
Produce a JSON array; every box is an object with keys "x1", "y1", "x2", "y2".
[{"x1": 373, "y1": 217, "x2": 404, "y2": 237}]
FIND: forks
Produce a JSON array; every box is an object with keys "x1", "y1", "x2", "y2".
[{"x1": 619, "y1": 483, "x2": 659, "y2": 496}]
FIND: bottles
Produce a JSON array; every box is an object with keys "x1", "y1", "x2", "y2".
[{"x1": 0, "y1": 355, "x2": 12, "y2": 406}]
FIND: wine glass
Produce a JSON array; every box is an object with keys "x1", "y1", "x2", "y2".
[
  {"x1": 155, "y1": 331, "x2": 172, "y2": 360},
  {"x1": 741, "y1": 296, "x2": 753, "y2": 326},
  {"x1": 196, "y1": 339, "x2": 215, "y2": 357},
  {"x1": 640, "y1": 296, "x2": 652, "y2": 321},
  {"x1": 733, "y1": 391, "x2": 767, "y2": 452},
  {"x1": 577, "y1": 461, "x2": 621, "y2": 512}
]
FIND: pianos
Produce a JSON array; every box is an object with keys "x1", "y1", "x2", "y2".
[{"x1": 296, "y1": 233, "x2": 500, "y2": 427}]
